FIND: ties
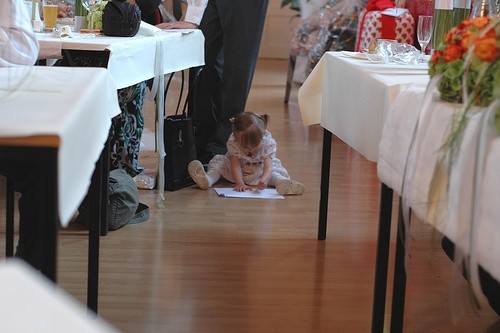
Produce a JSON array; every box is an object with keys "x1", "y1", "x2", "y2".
[{"x1": 173, "y1": 0, "x2": 182, "y2": 21}]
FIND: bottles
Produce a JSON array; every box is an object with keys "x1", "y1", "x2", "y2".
[{"x1": 470, "y1": 0, "x2": 497, "y2": 19}]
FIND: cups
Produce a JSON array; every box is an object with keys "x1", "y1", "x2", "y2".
[{"x1": 42, "y1": 0, "x2": 58, "y2": 29}]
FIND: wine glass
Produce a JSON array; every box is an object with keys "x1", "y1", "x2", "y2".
[
  {"x1": 416, "y1": 16, "x2": 433, "y2": 59},
  {"x1": 80, "y1": 0, "x2": 102, "y2": 32}
]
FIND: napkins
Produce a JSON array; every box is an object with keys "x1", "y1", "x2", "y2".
[{"x1": 55, "y1": 24, "x2": 74, "y2": 39}]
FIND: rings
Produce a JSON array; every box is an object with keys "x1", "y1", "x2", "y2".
[{"x1": 170, "y1": 23, "x2": 173, "y2": 26}]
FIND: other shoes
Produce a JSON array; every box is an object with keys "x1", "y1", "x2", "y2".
[
  {"x1": 188, "y1": 160, "x2": 211, "y2": 190},
  {"x1": 276, "y1": 179, "x2": 304, "y2": 195}
]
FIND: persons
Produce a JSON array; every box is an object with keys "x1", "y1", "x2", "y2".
[
  {"x1": 50, "y1": 0, "x2": 162, "y2": 192},
  {"x1": 0, "y1": 0, "x2": 59, "y2": 287},
  {"x1": 188, "y1": 111, "x2": 306, "y2": 196},
  {"x1": 154, "y1": 0, "x2": 270, "y2": 187}
]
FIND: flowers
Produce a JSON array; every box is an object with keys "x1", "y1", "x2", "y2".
[{"x1": 427, "y1": 14, "x2": 500, "y2": 108}]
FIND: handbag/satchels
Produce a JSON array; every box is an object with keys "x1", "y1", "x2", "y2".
[
  {"x1": 103, "y1": 1, "x2": 141, "y2": 37},
  {"x1": 165, "y1": 68, "x2": 204, "y2": 193},
  {"x1": 108, "y1": 169, "x2": 149, "y2": 231}
]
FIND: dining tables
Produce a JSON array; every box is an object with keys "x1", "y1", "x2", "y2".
[
  {"x1": 0, "y1": 64, "x2": 123, "y2": 320},
  {"x1": 31, "y1": 27, "x2": 206, "y2": 212},
  {"x1": 377, "y1": 82, "x2": 500, "y2": 333},
  {"x1": 297, "y1": 50, "x2": 431, "y2": 333}
]
